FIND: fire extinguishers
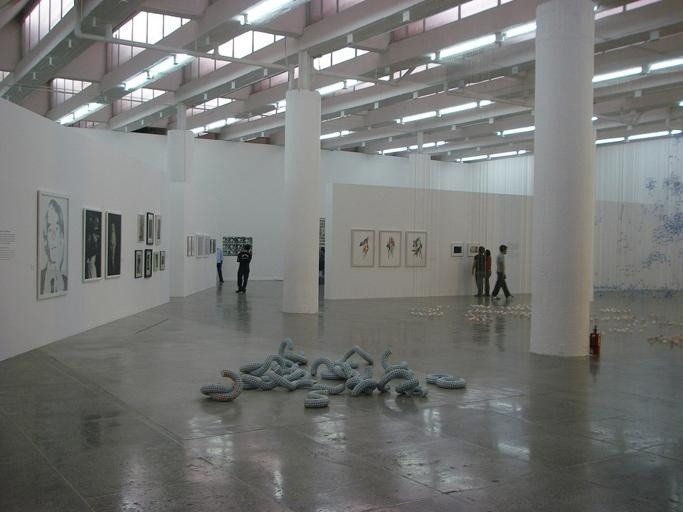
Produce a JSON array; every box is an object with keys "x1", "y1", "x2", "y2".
[{"x1": 589, "y1": 326, "x2": 600, "y2": 358}]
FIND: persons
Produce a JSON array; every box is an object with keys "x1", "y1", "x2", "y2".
[
  {"x1": 216, "y1": 247, "x2": 224, "y2": 283},
  {"x1": 235, "y1": 244, "x2": 252, "y2": 293},
  {"x1": 482, "y1": 249, "x2": 491, "y2": 296},
  {"x1": 491, "y1": 245, "x2": 514, "y2": 299},
  {"x1": 472, "y1": 246, "x2": 486, "y2": 297},
  {"x1": 38, "y1": 199, "x2": 66, "y2": 295}
]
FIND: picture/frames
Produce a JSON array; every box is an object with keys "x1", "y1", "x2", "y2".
[
  {"x1": 378, "y1": 229, "x2": 400, "y2": 267},
  {"x1": 404, "y1": 230, "x2": 427, "y2": 267},
  {"x1": 350, "y1": 229, "x2": 374, "y2": 269},
  {"x1": 187, "y1": 234, "x2": 217, "y2": 260},
  {"x1": 467, "y1": 242, "x2": 479, "y2": 258},
  {"x1": 34, "y1": 189, "x2": 168, "y2": 302},
  {"x1": 450, "y1": 243, "x2": 464, "y2": 257}
]
